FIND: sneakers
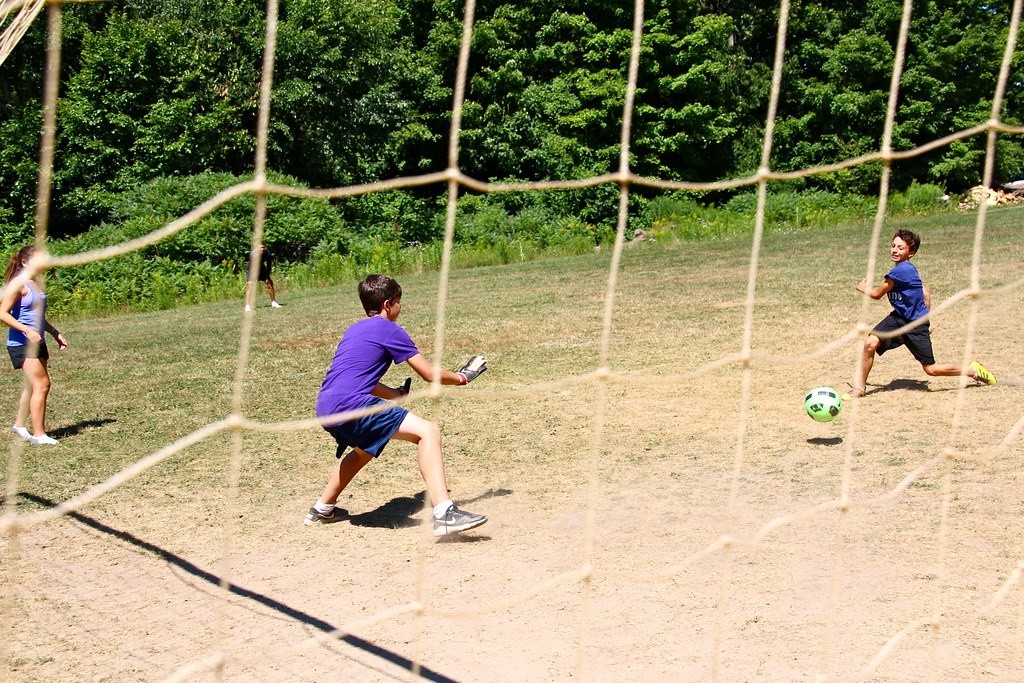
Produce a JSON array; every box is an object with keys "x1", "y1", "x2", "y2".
[
  {"x1": 244, "y1": 304, "x2": 252, "y2": 312},
  {"x1": 841, "y1": 385, "x2": 867, "y2": 401},
  {"x1": 432, "y1": 504, "x2": 488, "y2": 537},
  {"x1": 272, "y1": 300, "x2": 282, "y2": 308},
  {"x1": 10, "y1": 424, "x2": 32, "y2": 441},
  {"x1": 968, "y1": 360, "x2": 997, "y2": 387},
  {"x1": 303, "y1": 507, "x2": 349, "y2": 527},
  {"x1": 31, "y1": 434, "x2": 59, "y2": 446}
]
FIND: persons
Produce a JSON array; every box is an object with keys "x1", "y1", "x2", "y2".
[
  {"x1": 305, "y1": 274, "x2": 487, "y2": 537},
  {"x1": 245, "y1": 242, "x2": 283, "y2": 312},
  {"x1": 841, "y1": 229, "x2": 997, "y2": 400},
  {"x1": 0, "y1": 245, "x2": 66, "y2": 445}
]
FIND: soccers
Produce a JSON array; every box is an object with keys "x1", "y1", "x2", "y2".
[{"x1": 804, "y1": 388, "x2": 843, "y2": 421}]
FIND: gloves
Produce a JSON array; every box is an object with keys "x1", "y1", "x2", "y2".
[
  {"x1": 397, "y1": 377, "x2": 411, "y2": 398},
  {"x1": 457, "y1": 356, "x2": 487, "y2": 386}
]
